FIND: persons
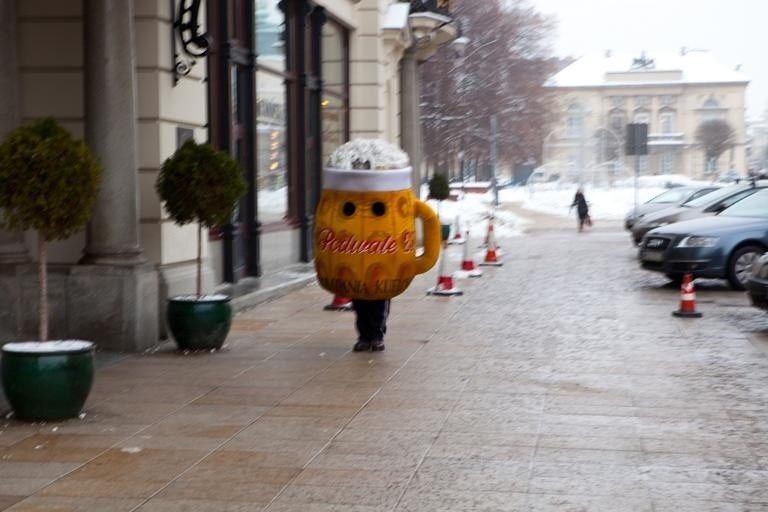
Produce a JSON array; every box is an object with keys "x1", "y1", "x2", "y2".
[{"x1": 572, "y1": 187, "x2": 592, "y2": 232}]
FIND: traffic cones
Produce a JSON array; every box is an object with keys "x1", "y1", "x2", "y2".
[
  {"x1": 426, "y1": 239, "x2": 464, "y2": 297},
  {"x1": 322, "y1": 290, "x2": 355, "y2": 311},
  {"x1": 477, "y1": 215, "x2": 501, "y2": 268},
  {"x1": 671, "y1": 267, "x2": 703, "y2": 320},
  {"x1": 449, "y1": 214, "x2": 465, "y2": 244},
  {"x1": 458, "y1": 228, "x2": 482, "y2": 280}
]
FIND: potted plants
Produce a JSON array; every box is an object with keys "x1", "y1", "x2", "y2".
[
  {"x1": 153, "y1": 138, "x2": 245, "y2": 353},
  {"x1": 428, "y1": 175, "x2": 449, "y2": 240},
  {"x1": 0, "y1": 118, "x2": 95, "y2": 423}
]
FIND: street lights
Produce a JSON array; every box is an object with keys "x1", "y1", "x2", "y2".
[{"x1": 630, "y1": 106, "x2": 643, "y2": 207}]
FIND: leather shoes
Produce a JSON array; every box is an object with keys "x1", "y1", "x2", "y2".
[
  {"x1": 370, "y1": 340, "x2": 386, "y2": 351},
  {"x1": 354, "y1": 339, "x2": 371, "y2": 351}
]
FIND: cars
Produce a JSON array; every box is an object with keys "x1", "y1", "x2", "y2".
[
  {"x1": 623, "y1": 173, "x2": 766, "y2": 292},
  {"x1": 743, "y1": 251, "x2": 767, "y2": 309}
]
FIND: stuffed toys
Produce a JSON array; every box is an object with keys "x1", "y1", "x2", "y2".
[{"x1": 313, "y1": 137, "x2": 442, "y2": 352}]
